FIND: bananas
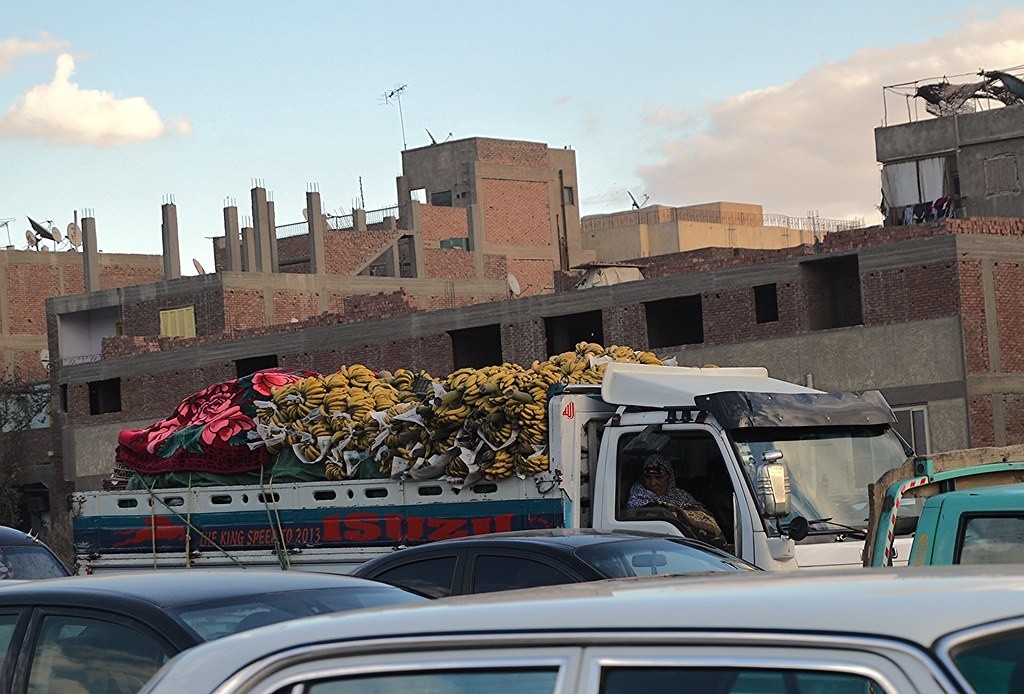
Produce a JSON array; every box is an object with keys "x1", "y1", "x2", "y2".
[{"x1": 255, "y1": 341, "x2": 719, "y2": 481}]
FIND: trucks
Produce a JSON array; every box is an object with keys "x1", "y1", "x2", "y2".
[
  {"x1": 724, "y1": 445, "x2": 1023, "y2": 693},
  {"x1": 69, "y1": 362, "x2": 937, "y2": 578}
]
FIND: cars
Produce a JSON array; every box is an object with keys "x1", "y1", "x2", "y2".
[
  {"x1": 348, "y1": 526, "x2": 767, "y2": 602},
  {"x1": 0, "y1": 523, "x2": 91, "y2": 658},
  {"x1": 133, "y1": 566, "x2": 1024, "y2": 694},
  {"x1": 0, "y1": 569, "x2": 436, "y2": 694}
]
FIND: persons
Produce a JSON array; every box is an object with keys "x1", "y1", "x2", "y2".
[{"x1": 625, "y1": 454, "x2": 735, "y2": 555}]
